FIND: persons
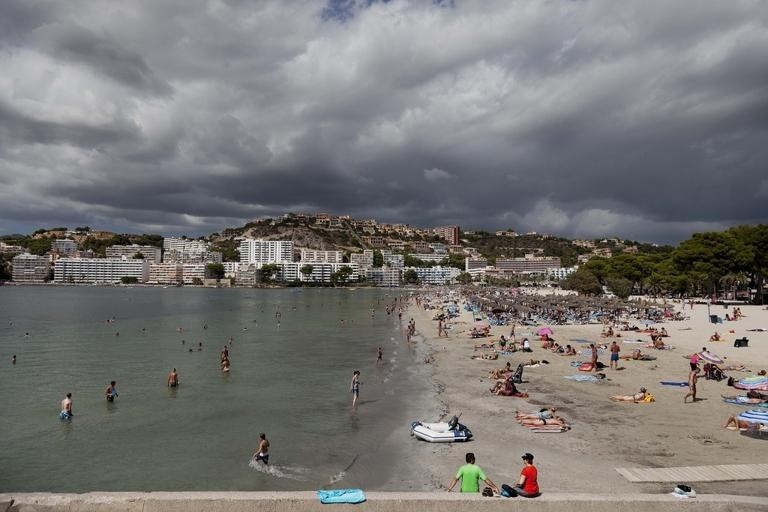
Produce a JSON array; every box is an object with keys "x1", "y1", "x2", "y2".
[
  {"x1": 445, "y1": 452, "x2": 499, "y2": 494},
  {"x1": 251, "y1": 432, "x2": 269, "y2": 466},
  {"x1": 106, "y1": 312, "x2": 281, "y2": 374},
  {"x1": 9, "y1": 321, "x2": 30, "y2": 365},
  {"x1": 59, "y1": 393, "x2": 73, "y2": 420},
  {"x1": 350, "y1": 284, "x2": 768, "y2": 433},
  {"x1": 167, "y1": 368, "x2": 179, "y2": 388},
  {"x1": 513, "y1": 453, "x2": 539, "y2": 498},
  {"x1": 105, "y1": 380, "x2": 119, "y2": 402}
]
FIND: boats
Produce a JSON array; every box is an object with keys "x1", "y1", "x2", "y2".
[{"x1": 411, "y1": 415, "x2": 472, "y2": 443}]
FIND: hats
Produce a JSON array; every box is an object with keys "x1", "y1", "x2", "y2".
[{"x1": 522, "y1": 453, "x2": 533, "y2": 459}]
